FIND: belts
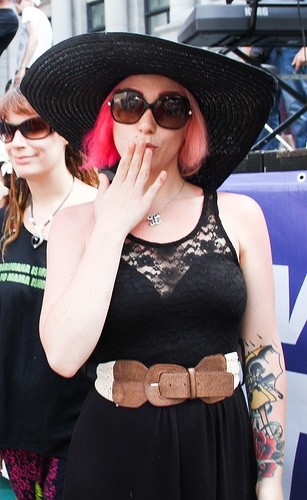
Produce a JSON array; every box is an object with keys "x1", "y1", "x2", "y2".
[{"x1": 93, "y1": 351, "x2": 246, "y2": 409}]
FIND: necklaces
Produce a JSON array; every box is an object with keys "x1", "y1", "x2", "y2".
[
  {"x1": 29, "y1": 174, "x2": 74, "y2": 249},
  {"x1": 144, "y1": 179, "x2": 185, "y2": 225}
]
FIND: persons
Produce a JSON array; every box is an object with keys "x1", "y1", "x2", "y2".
[
  {"x1": 240, "y1": 42, "x2": 307, "y2": 149},
  {"x1": 0, "y1": 0, "x2": 103, "y2": 500},
  {"x1": 20, "y1": 33, "x2": 288, "y2": 500}
]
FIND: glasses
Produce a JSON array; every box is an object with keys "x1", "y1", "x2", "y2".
[
  {"x1": 104, "y1": 88, "x2": 195, "y2": 132},
  {"x1": 0, "y1": 118, "x2": 54, "y2": 143}
]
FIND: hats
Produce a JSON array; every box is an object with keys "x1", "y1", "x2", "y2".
[{"x1": 19, "y1": 30, "x2": 280, "y2": 195}]
